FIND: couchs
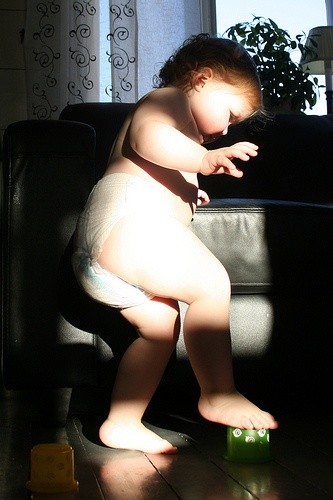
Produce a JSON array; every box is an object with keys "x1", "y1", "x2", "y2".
[{"x1": 0, "y1": 103, "x2": 333, "y2": 389}]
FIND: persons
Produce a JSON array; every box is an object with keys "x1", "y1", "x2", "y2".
[{"x1": 72, "y1": 32, "x2": 278, "y2": 453}]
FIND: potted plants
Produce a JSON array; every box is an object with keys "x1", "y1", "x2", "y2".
[{"x1": 222, "y1": 16, "x2": 325, "y2": 114}]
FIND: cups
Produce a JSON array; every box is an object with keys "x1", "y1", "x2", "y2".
[{"x1": 226, "y1": 426, "x2": 270, "y2": 464}]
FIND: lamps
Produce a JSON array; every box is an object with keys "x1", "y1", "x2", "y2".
[{"x1": 300, "y1": 26, "x2": 333, "y2": 116}]
